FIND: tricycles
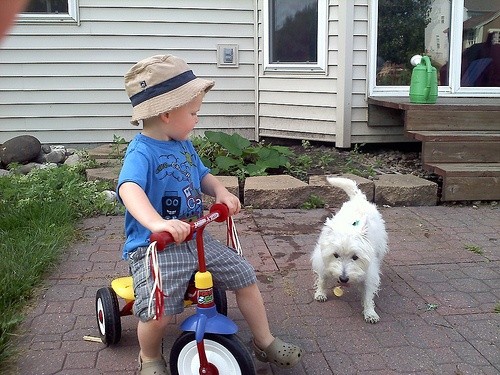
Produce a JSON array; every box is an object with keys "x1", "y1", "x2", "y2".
[{"x1": 95, "y1": 201, "x2": 257, "y2": 375}]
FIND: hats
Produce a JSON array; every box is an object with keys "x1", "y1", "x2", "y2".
[{"x1": 124, "y1": 54, "x2": 215, "y2": 126}]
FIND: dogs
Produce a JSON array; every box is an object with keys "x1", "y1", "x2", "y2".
[{"x1": 311, "y1": 176, "x2": 389, "y2": 323}]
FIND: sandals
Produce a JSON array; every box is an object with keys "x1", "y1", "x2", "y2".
[
  {"x1": 137, "y1": 353, "x2": 170, "y2": 375},
  {"x1": 252, "y1": 336, "x2": 304, "y2": 369}
]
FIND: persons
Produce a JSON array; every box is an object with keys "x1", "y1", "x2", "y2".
[{"x1": 119, "y1": 56, "x2": 305, "y2": 375}]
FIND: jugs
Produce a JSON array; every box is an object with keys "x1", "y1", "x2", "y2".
[{"x1": 408, "y1": 55, "x2": 439, "y2": 105}]
